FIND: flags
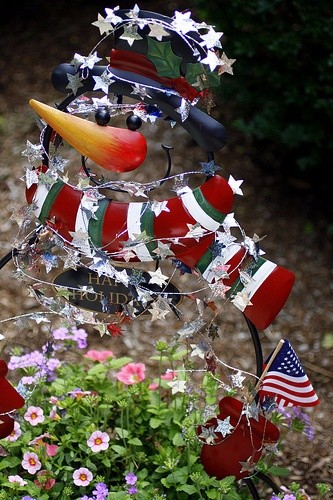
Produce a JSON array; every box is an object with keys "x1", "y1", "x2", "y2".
[{"x1": 257, "y1": 339, "x2": 320, "y2": 406}]
